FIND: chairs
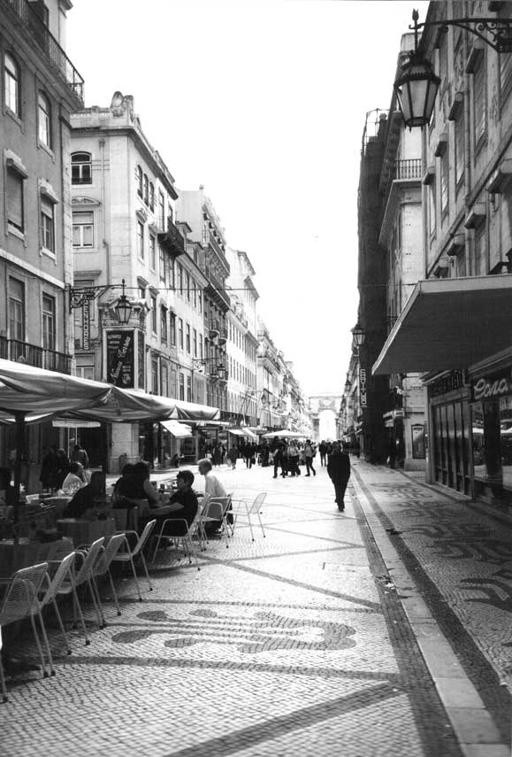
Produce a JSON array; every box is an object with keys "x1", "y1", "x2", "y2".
[
  {"x1": 1, "y1": 486, "x2": 121, "y2": 699},
  {"x1": 100, "y1": 486, "x2": 269, "y2": 600}
]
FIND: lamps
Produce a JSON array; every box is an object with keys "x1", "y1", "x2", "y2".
[
  {"x1": 193, "y1": 353, "x2": 228, "y2": 383},
  {"x1": 66, "y1": 277, "x2": 134, "y2": 324},
  {"x1": 389, "y1": 4, "x2": 511, "y2": 129}
]
